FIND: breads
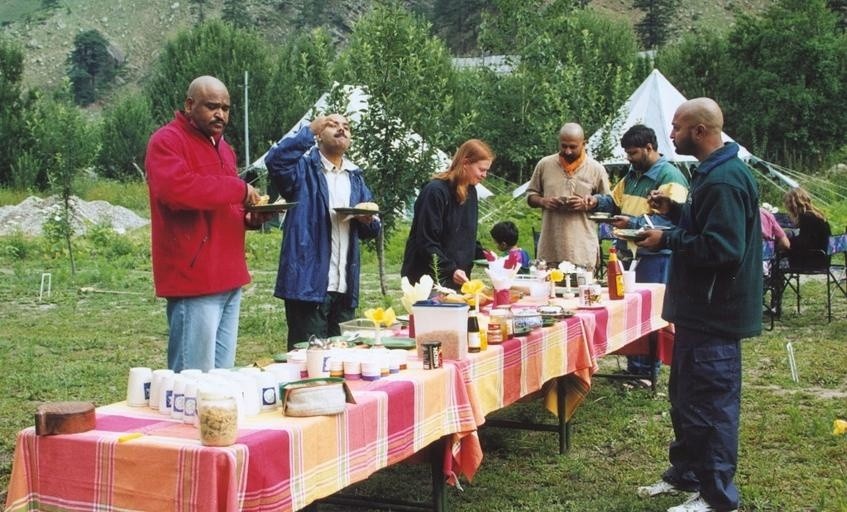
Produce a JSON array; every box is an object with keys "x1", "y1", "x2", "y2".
[{"x1": 355, "y1": 202, "x2": 380, "y2": 211}]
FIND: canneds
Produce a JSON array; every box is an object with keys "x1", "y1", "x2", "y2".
[
  {"x1": 422, "y1": 341, "x2": 443, "y2": 370},
  {"x1": 579, "y1": 285, "x2": 591, "y2": 307},
  {"x1": 487, "y1": 304, "x2": 514, "y2": 344}
]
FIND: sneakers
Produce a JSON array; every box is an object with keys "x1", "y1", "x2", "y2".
[
  {"x1": 638, "y1": 475, "x2": 703, "y2": 497},
  {"x1": 763, "y1": 309, "x2": 779, "y2": 317},
  {"x1": 665, "y1": 483, "x2": 741, "y2": 512}
]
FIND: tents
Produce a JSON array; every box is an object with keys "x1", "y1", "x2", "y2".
[
  {"x1": 251, "y1": 79, "x2": 494, "y2": 216},
  {"x1": 509, "y1": 66, "x2": 754, "y2": 200}
]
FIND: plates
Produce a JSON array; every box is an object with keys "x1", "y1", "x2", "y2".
[
  {"x1": 610, "y1": 228, "x2": 648, "y2": 243},
  {"x1": 242, "y1": 200, "x2": 300, "y2": 214},
  {"x1": 515, "y1": 299, "x2": 607, "y2": 319},
  {"x1": 291, "y1": 334, "x2": 418, "y2": 348},
  {"x1": 333, "y1": 207, "x2": 382, "y2": 216},
  {"x1": 470, "y1": 258, "x2": 489, "y2": 268},
  {"x1": 586, "y1": 210, "x2": 619, "y2": 222}
]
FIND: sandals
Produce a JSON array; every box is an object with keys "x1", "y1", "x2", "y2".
[{"x1": 607, "y1": 370, "x2": 660, "y2": 391}]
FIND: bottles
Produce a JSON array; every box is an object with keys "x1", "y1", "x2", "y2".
[
  {"x1": 467, "y1": 299, "x2": 482, "y2": 354},
  {"x1": 606, "y1": 246, "x2": 627, "y2": 301}
]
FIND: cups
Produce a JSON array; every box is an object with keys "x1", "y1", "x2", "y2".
[
  {"x1": 579, "y1": 282, "x2": 602, "y2": 306},
  {"x1": 125, "y1": 347, "x2": 414, "y2": 431}
]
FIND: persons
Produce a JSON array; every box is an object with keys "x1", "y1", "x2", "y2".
[
  {"x1": 524, "y1": 120, "x2": 611, "y2": 275},
  {"x1": 757, "y1": 206, "x2": 792, "y2": 283},
  {"x1": 633, "y1": 96, "x2": 765, "y2": 512},
  {"x1": 481, "y1": 220, "x2": 528, "y2": 269},
  {"x1": 261, "y1": 113, "x2": 382, "y2": 354},
  {"x1": 143, "y1": 75, "x2": 274, "y2": 374},
  {"x1": 579, "y1": 123, "x2": 690, "y2": 392},
  {"x1": 762, "y1": 186, "x2": 832, "y2": 321},
  {"x1": 399, "y1": 139, "x2": 495, "y2": 294}
]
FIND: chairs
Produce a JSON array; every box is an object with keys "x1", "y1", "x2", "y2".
[
  {"x1": 532, "y1": 227, "x2": 540, "y2": 259},
  {"x1": 598, "y1": 221, "x2": 617, "y2": 279},
  {"x1": 783, "y1": 226, "x2": 847, "y2": 322},
  {"x1": 761, "y1": 239, "x2": 778, "y2": 332}
]
